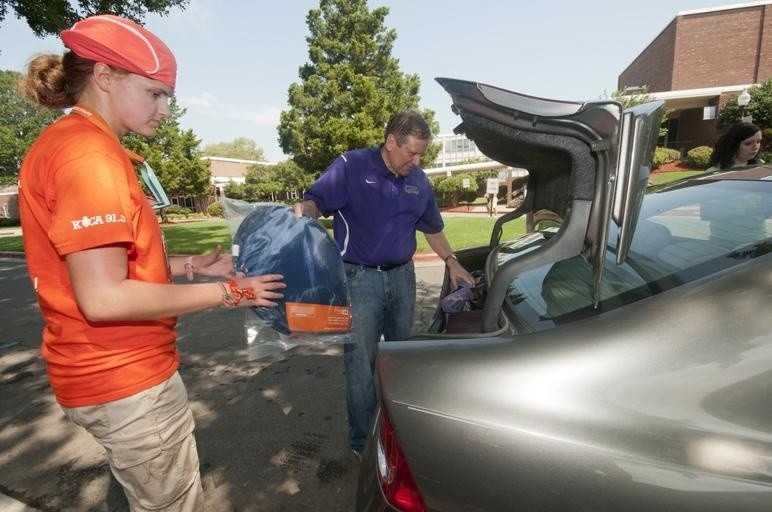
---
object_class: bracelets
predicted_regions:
[214,278,256,308]
[184,255,196,282]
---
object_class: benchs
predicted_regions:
[630,221,729,274]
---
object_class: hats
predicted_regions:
[56,14,177,89]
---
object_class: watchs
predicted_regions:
[444,253,458,269]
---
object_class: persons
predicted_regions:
[292,109,476,457]
[483,192,498,217]
[701,121,771,245]
[16,13,288,512]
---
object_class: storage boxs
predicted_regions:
[619,2,772,91]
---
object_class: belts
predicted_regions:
[339,258,414,273]
